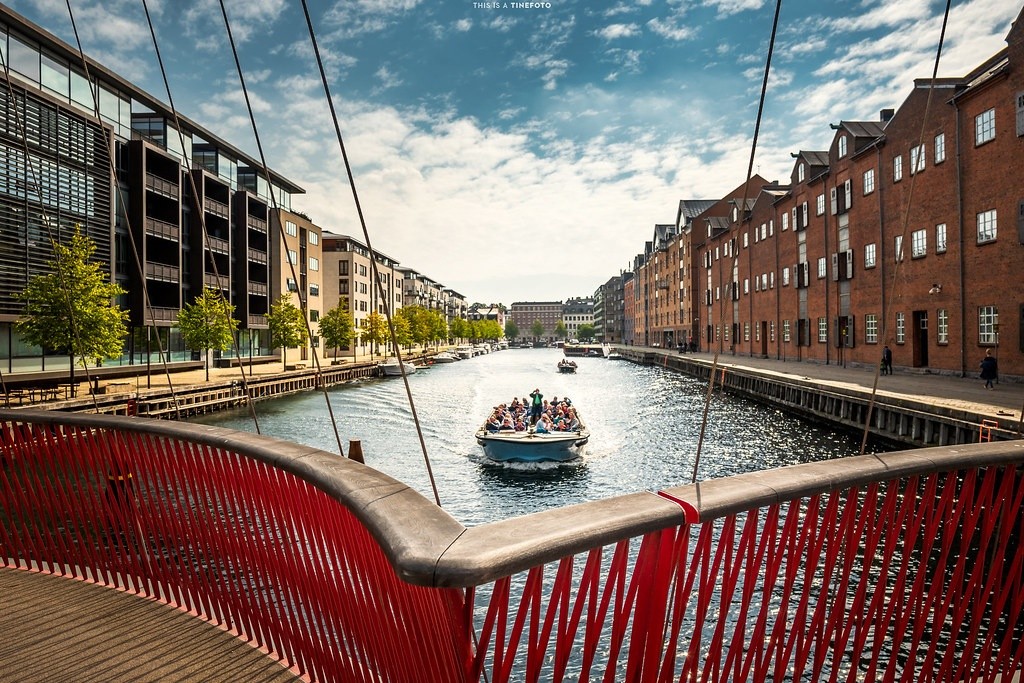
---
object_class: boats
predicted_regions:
[608,353,622,360]
[475,402,590,463]
[433,351,461,363]
[584,349,602,356]
[518,342,533,348]
[558,363,577,373]
[454,341,508,359]
[376,357,416,375]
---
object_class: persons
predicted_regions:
[420,334,699,436]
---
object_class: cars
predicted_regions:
[569,338,580,345]
